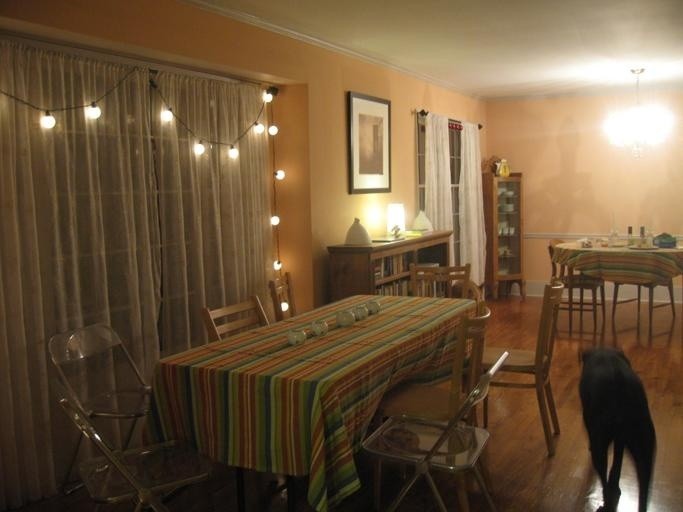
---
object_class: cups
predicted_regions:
[497,224,516,236]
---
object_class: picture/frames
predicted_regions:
[348,90,392,194]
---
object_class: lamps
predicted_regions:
[387,202,404,234]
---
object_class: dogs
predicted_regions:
[577,344,656,512]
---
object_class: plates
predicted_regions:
[611,242,626,246]
[501,204,515,213]
[630,245,660,250]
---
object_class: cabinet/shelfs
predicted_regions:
[483,172,525,302]
[327,231,452,301]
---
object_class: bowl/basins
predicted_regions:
[502,191,514,196]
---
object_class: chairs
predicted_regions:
[46,324,209,512]
[360,350,509,512]
[268,268,297,320]
[546,243,605,328]
[481,276,561,457]
[201,294,270,341]
[611,279,676,326]
[408,262,471,297]
[375,306,491,486]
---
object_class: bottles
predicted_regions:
[343,218,372,246]
[287,300,381,345]
[626,225,646,248]
[411,210,433,230]
[498,158,510,178]
